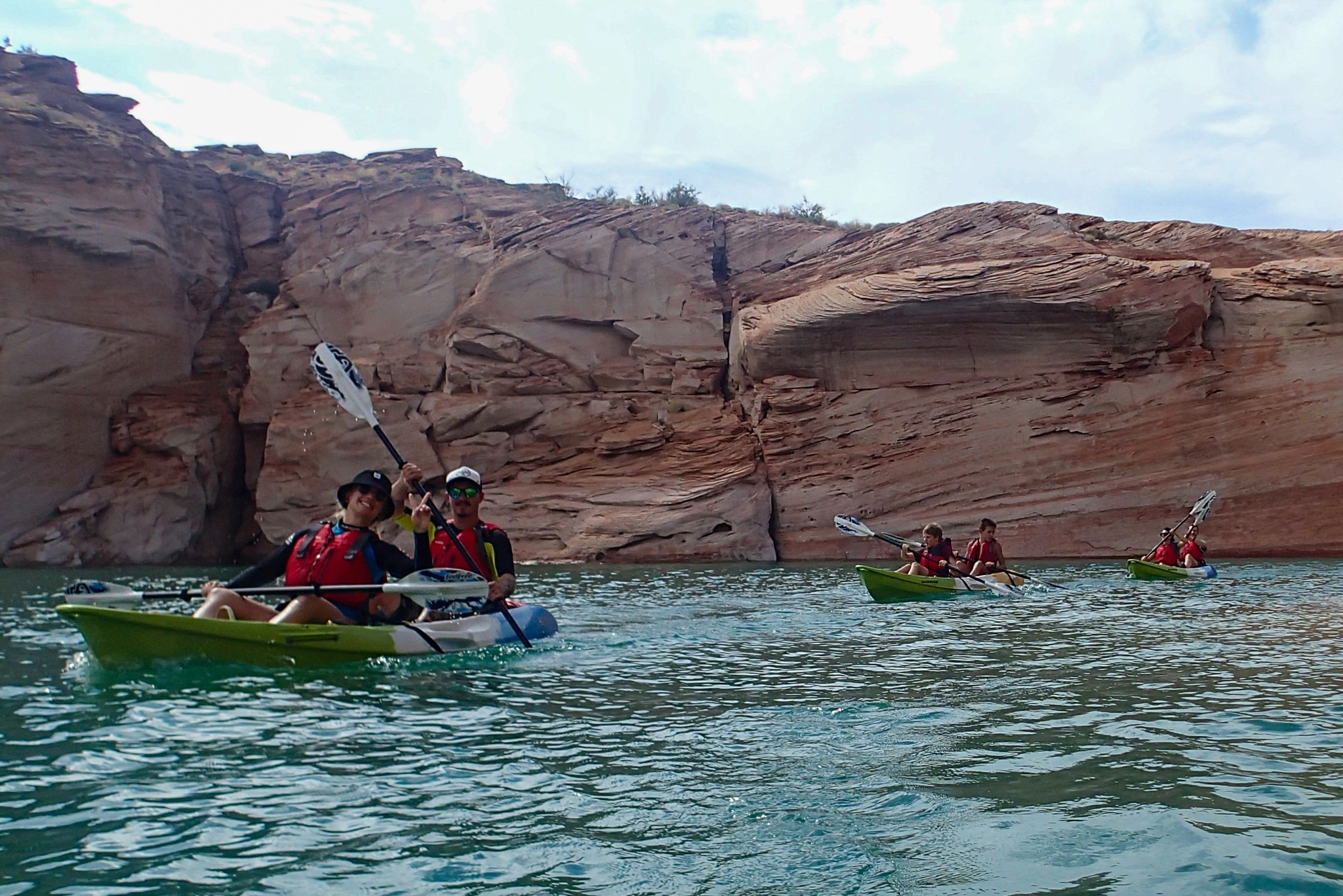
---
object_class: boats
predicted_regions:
[56,602,559,674]
[855,564,1025,604]
[1126,559,1218,581]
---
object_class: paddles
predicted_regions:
[1145,489,1217,558]
[833,514,1024,598]
[311,343,534,649]
[880,531,1070,591]
[1186,507,1211,537]
[64,567,488,615]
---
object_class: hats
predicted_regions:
[337,469,395,522]
[445,466,483,493]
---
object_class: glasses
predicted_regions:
[447,487,479,499]
[356,484,387,502]
[1160,532,1169,536]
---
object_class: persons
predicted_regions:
[1141,524,1207,568]
[368,462,516,624]
[193,470,431,625]
[895,518,1006,577]
